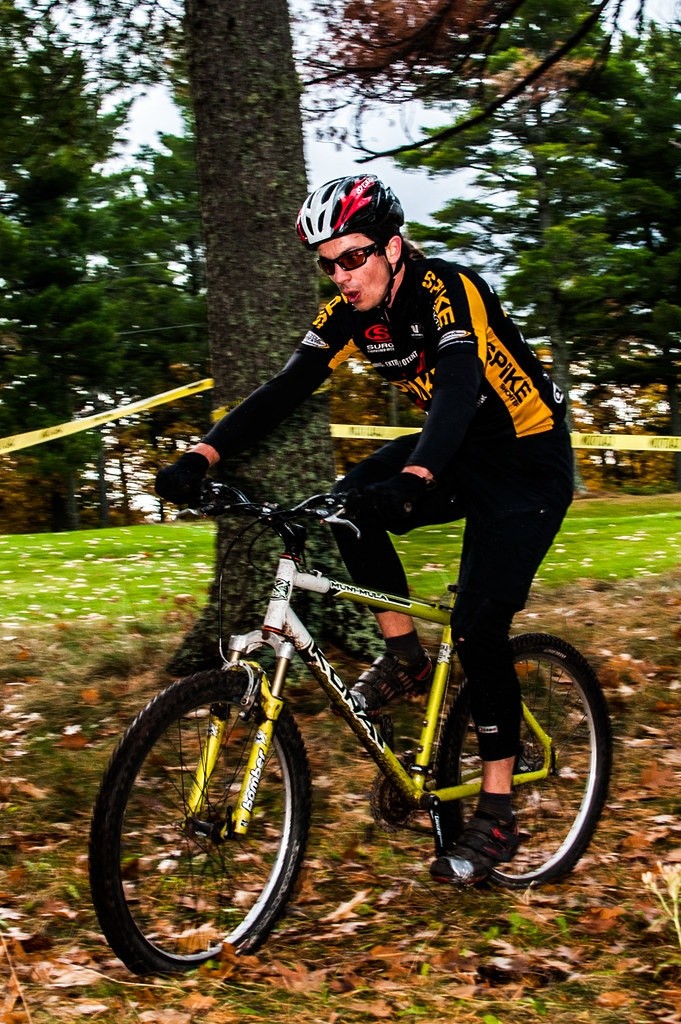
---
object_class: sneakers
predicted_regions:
[330,647,433,718]
[428,807,519,885]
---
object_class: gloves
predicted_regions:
[361,473,428,534]
[154,452,209,510]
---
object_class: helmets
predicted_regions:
[295,174,404,251]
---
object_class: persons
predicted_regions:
[155,174,573,883]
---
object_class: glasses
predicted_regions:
[317,243,377,275]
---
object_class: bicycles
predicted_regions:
[85,479,615,979]
